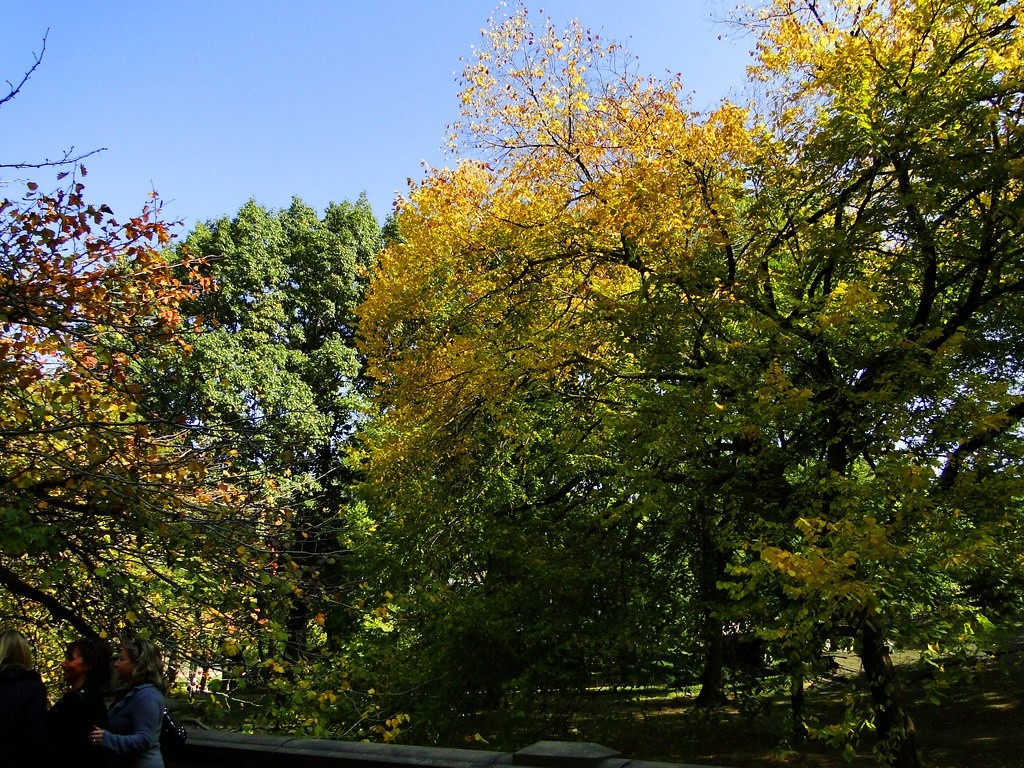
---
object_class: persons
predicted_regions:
[0,630,46,768]
[89,639,165,768]
[47,638,113,768]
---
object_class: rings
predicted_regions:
[93,739,95,742]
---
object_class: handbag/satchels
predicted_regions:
[159,709,187,750]
